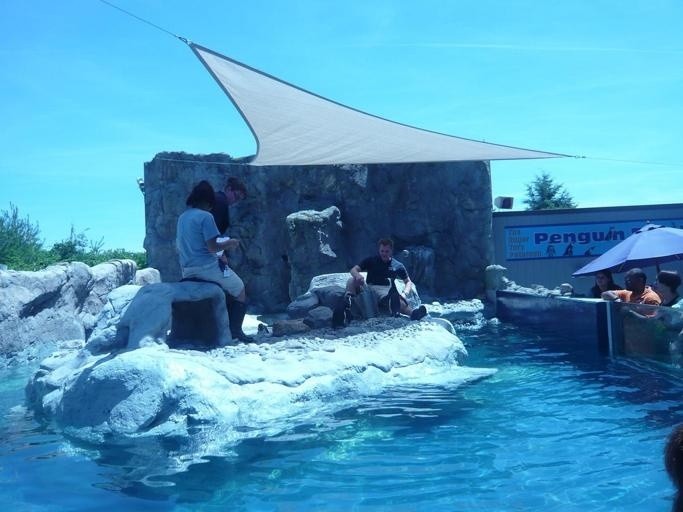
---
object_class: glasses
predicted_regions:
[234,191,240,200]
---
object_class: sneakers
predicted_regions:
[411,305,426,320]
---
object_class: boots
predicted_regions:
[228,300,253,342]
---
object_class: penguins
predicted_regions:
[332,290,358,330]
[256,323,270,335]
[410,304,427,321]
[380,276,409,318]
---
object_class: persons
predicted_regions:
[333,239,426,330]
[599,267,661,355]
[575,269,624,370]
[208,176,249,299]
[620,270,683,370]
[175,179,256,344]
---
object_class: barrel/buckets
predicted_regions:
[352,282,380,319]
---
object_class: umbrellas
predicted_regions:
[570,220,683,278]
